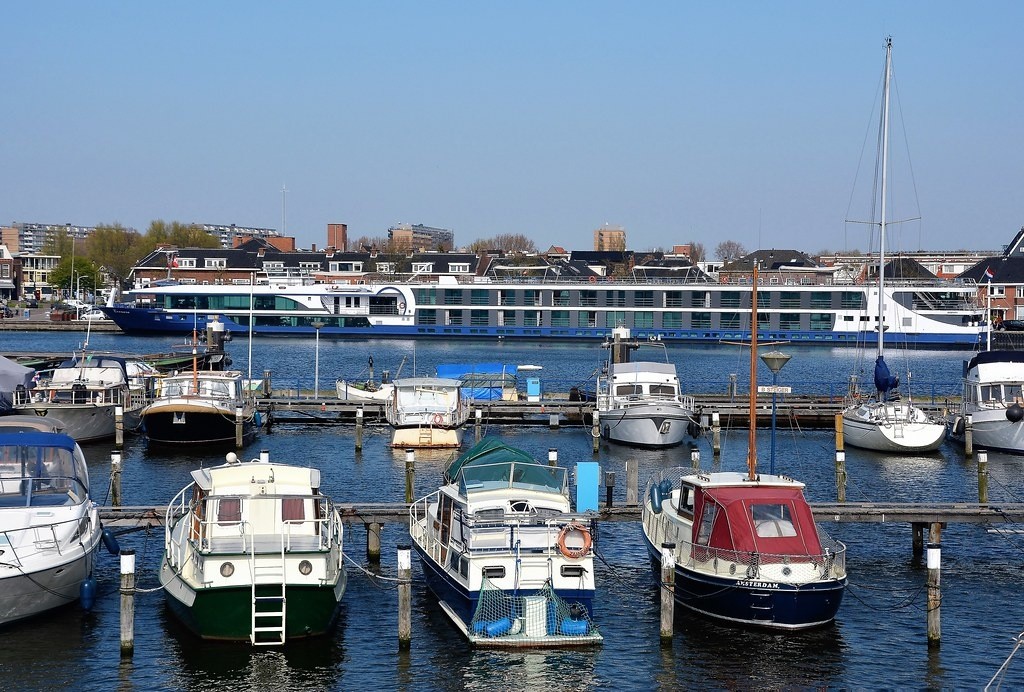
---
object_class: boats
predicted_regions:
[640,463,850,628]
[0,410,105,626]
[410,433,604,645]
[0,326,1023,462]
[154,448,348,649]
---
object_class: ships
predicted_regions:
[97,278,996,347]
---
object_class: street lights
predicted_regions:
[76,274,89,321]
[759,351,791,474]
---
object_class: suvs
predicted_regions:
[0,298,113,321]
[996,319,1024,331]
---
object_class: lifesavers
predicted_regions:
[399,302,406,309]
[589,276,596,284]
[434,414,443,425]
[606,276,614,283]
[722,276,728,283]
[558,522,592,559]
[855,277,864,285]
